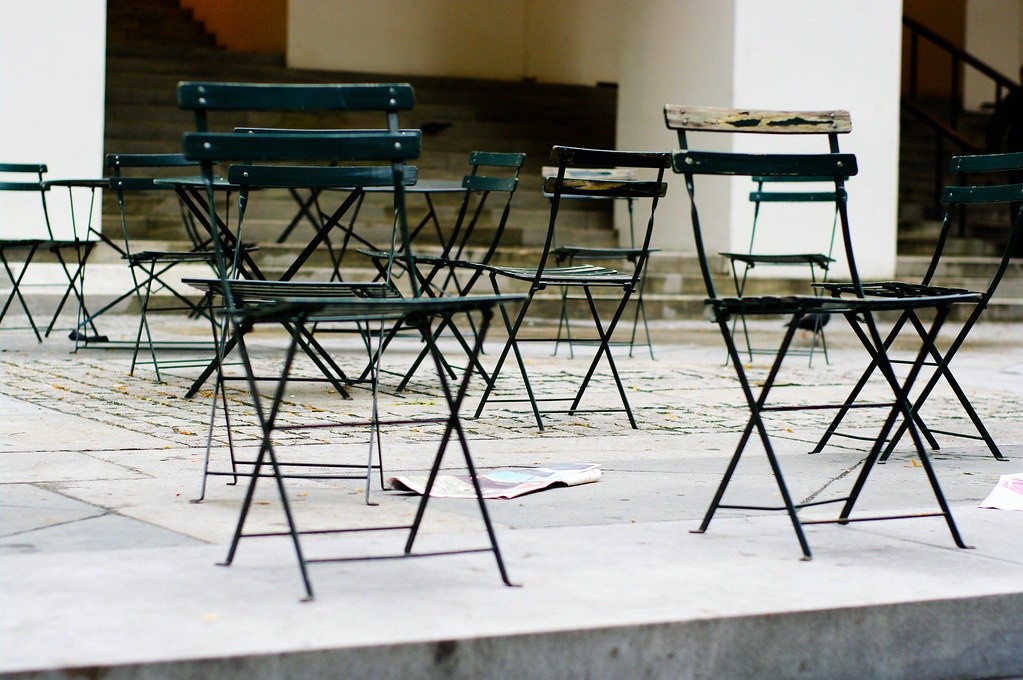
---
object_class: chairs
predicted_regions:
[0,80,1023,604]
